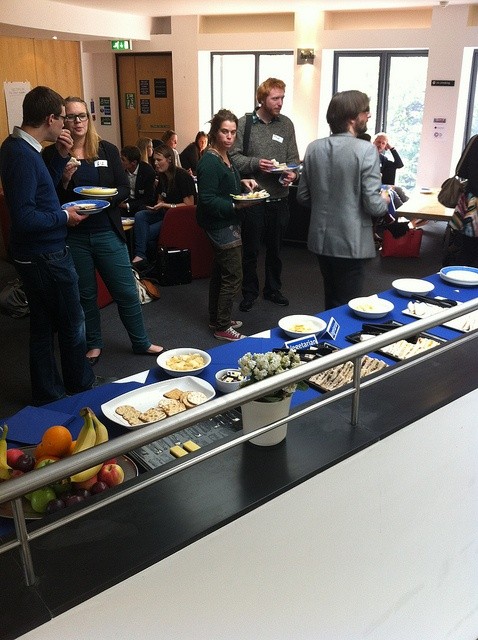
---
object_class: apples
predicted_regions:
[6,449,23,463]
[23,460,56,513]
[82,457,125,490]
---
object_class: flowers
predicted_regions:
[235,347,303,401]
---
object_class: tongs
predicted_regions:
[413,294,457,308]
[273,348,332,356]
[362,323,417,344]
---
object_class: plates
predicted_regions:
[290,341,342,393]
[0,446,138,521]
[440,266,478,286]
[278,315,327,340]
[156,348,211,376]
[273,166,300,173]
[348,297,393,320]
[100,376,216,432]
[127,409,243,470]
[74,186,117,200]
[402,296,478,333]
[122,219,135,226]
[391,278,435,298]
[62,199,110,215]
[346,320,448,362]
[234,192,268,202]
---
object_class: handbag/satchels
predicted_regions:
[438,134,478,207]
[379,227,423,259]
[142,280,161,300]
[149,247,192,285]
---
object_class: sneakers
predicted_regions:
[209,320,243,329]
[213,326,247,341]
[131,269,151,305]
[239,297,256,311]
[263,290,289,307]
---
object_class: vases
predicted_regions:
[240,395,291,447]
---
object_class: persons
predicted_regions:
[296,90,391,311]
[43,96,169,366]
[161,129,193,176]
[149,139,164,166]
[180,131,208,171]
[443,133,477,269]
[135,136,154,168]
[131,144,197,267]
[371,132,404,250]
[118,145,156,217]
[0,85,120,406]
[196,109,258,341]
[228,78,300,311]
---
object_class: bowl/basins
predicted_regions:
[215,369,244,392]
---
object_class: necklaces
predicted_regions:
[76,155,80,158]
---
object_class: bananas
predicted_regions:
[0,423,14,481]
[72,408,109,483]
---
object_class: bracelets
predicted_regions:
[170,203,177,209]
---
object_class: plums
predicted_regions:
[44,482,107,513]
[16,454,36,474]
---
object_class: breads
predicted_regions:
[381,336,440,360]
[308,355,392,392]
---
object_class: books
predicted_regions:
[380,184,409,213]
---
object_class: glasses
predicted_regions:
[52,113,68,123]
[66,113,89,122]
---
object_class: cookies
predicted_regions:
[169,444,185,460]
[184,439,199,453]
[113,389,206,426]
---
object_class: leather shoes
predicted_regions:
[85,347,102,365]
[134,345,168,356]
